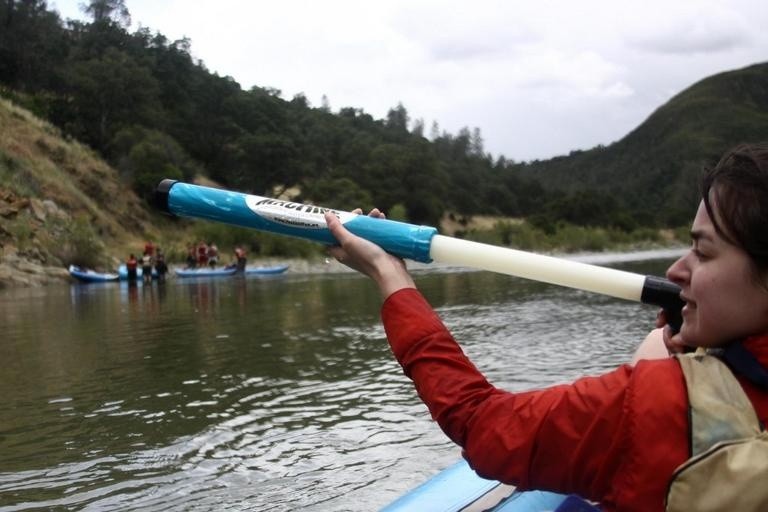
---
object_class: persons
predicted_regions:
[319,141,767,512]
[155,253,168,280]
[141,251,153,283]
[127,276,248,319]
[144,239,155,257]
[182,239,220,270]
[126,254,138,287]
[232,246,248,271]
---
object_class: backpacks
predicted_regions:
[665,348,767,512]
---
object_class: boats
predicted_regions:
[68,262,290,282]
